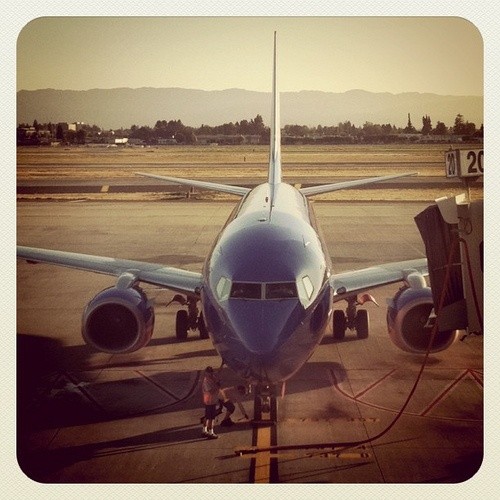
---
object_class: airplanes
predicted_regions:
[16,29,462,428]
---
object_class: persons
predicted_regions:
[200,367,221,439]
[200,383,250,427]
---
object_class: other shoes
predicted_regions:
[208,434,219,440]
[202,431,209,437]
[220,417,235,425]
